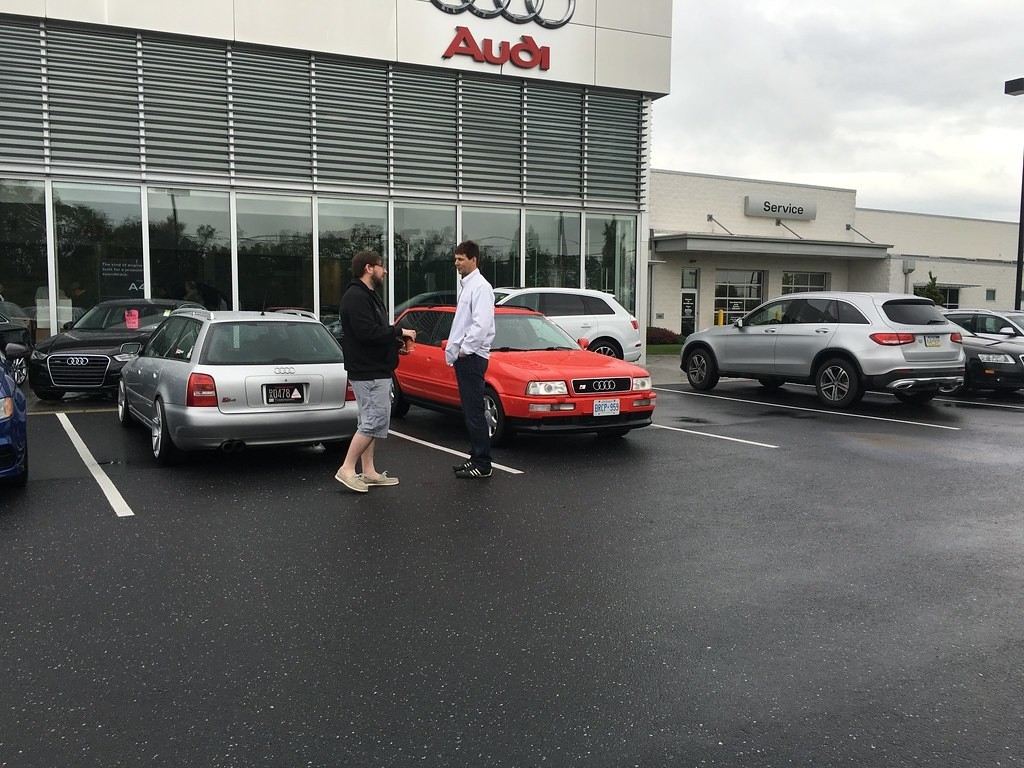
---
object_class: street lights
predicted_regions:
[1004,77,1024,312]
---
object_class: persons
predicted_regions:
[215,293,228,311]
[183,280,204,305]
[335,251,416,492]
[444,242,496,479]
[76,284,97,310]
[0,284,7,301]
[34,286,66,305]
[159,284,171,299]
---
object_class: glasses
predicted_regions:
[375,263,386,270]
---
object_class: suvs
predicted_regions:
[936,309,1024,398]
[490,287,642,366]
[681,291,968,410]
[28,299,209,401]
[116,309,361,462]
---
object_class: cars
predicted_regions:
[388,307,657,448]
[0,343,34,486]
[392,290,458,321]
[0,300,31,385]
[266,306,344,341]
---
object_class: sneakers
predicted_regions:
[335,467,368,493]
[453,458,472,471]
[455,465,492,479]
[360,470,399,486]
